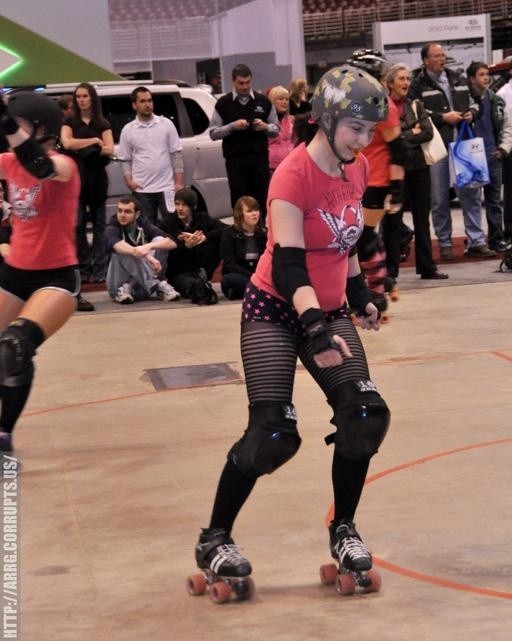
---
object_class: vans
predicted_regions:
[1,79,236,233]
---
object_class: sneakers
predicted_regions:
[155,279,181,302]
[400,229,512,261]
[81,275,90,284]
[114,283,134,304]
[93,276,105,283]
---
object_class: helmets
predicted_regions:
[310,64,389,123]
[345,47,390,82]
[6,90,63,139]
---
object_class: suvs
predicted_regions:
[487,55,512,94]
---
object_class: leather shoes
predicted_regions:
[420,268,449,280]
[77,294,95,310]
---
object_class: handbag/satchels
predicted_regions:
[409,99,448,168]
[447,117,490,191]
[188,274,219,305]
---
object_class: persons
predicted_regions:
[194,65,392,576]
[0,83,83,482]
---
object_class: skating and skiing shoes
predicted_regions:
[320,519,383,595]
[0,431,25,478]
[347,266,400,326]
[189,525,256,603]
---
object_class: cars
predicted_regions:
[444,56,465,74]
